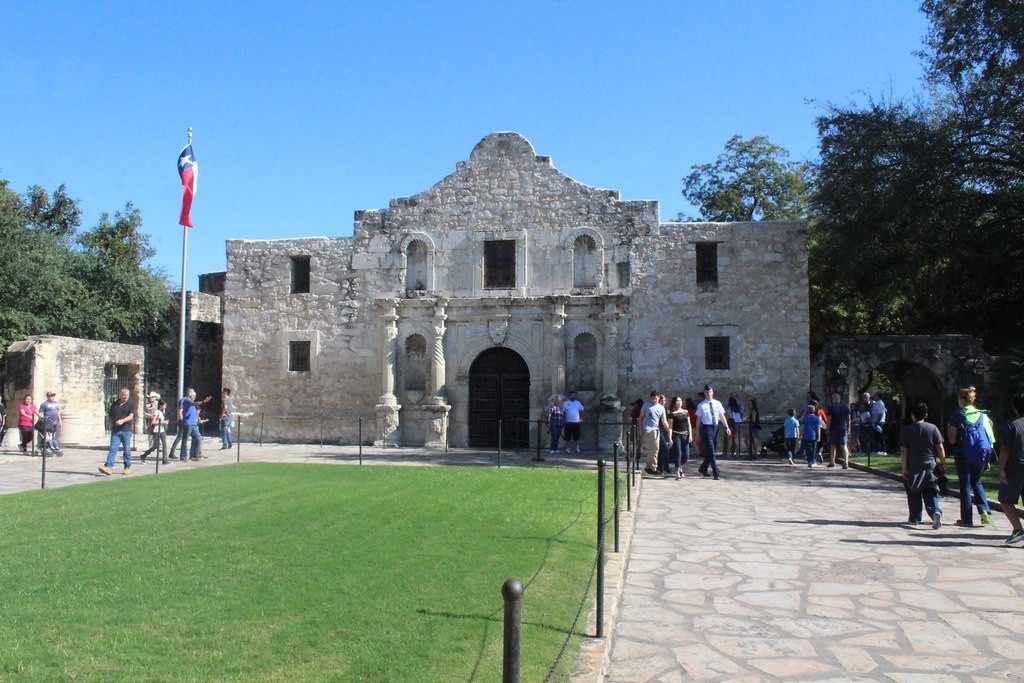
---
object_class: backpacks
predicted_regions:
[957,411,991,467]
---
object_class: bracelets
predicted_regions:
[725,426,729,429]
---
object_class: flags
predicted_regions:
[177,144,198,229]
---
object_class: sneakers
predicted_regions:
[956,519,973,526]
[1006,528,1024,544]
[980,514,991,524]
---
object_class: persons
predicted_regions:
[826,393,851,469]
[695,384,731,480]
[948,386,992,526]
[18,391,62,455]
[998,394,1024,543]
[799,405,821,467]
[795,391,827,463]
[784,408,800,466]
[140,391,169,465]
[899,403,946,530]
[684,390,762,459]
[0,396,6,433]
[169,387,212,462]
[630,390,672,476]
[850,390,887,457]
[547,391,584,454]
[667,396,693,481]
[99,387,134,475]
[219,388,232,450]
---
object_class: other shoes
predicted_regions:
[228,445,233,449]
[908,520,919,524]
[98,466,112,475]
[18,444,24,451]
[932,511,942,530]
[140,455,147,464]
[566,448,570,453]
[180,458,191,462]
[219,447,228,451]
[576,447,581,453]
[169,453,178,459]
[550,450,555,453]
[644,451,896,480]
[23,451,30,456]
[189,456,205,461]
[555,449,560,453]
[123,469,130,475]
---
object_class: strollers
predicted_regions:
[761,426,788,458]
[34,418,65,458]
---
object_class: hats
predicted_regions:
[703,384,712,390]
[146,391,160,399]
[46,391,56,398]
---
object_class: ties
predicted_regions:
[710,402,716,427]
